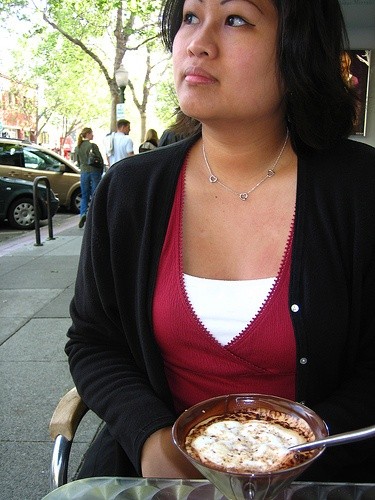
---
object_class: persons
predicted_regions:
[139,129,159,152]
[339,52,360,128]
[74,127,103,228]
[62,0,375,484]
[104,119,133,169]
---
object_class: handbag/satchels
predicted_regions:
[87,143,101,168]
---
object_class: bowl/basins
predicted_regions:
[171,393,328,500]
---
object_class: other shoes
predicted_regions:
[79,214,87,228]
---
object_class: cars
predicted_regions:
[0,175,61,230]
[0,137,91,214]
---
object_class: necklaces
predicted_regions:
[202,126,289,200]
